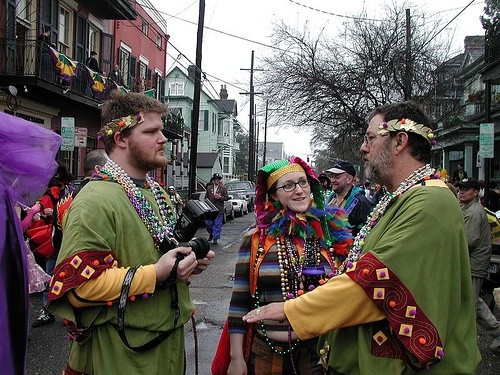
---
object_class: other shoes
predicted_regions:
[208,236,212,241]
[214,240,217,244]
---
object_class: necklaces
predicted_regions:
[338,163,435,277]
[103,158,177,253]
[255,233,337,375]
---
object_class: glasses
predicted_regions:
[363,133,378,146]
[458,186,469,191]
[274,179,310,192]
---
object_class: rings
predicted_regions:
[257,311,260,314]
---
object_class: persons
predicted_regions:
[321,160,387,235]
[243,104,472,375]
[441,160,500,359]
[48,90,217,375]
[226,158,355,375]
[0,26,127,375]
[169,173,229,244]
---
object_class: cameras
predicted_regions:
[158,198,219,260]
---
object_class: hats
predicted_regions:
[317,174,331,186]
[326,161,356,177]
[454,177,479,192]
[211,173,222,180]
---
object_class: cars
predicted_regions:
[188,179,256,224]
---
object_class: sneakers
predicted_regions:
[32,312,54,327]
